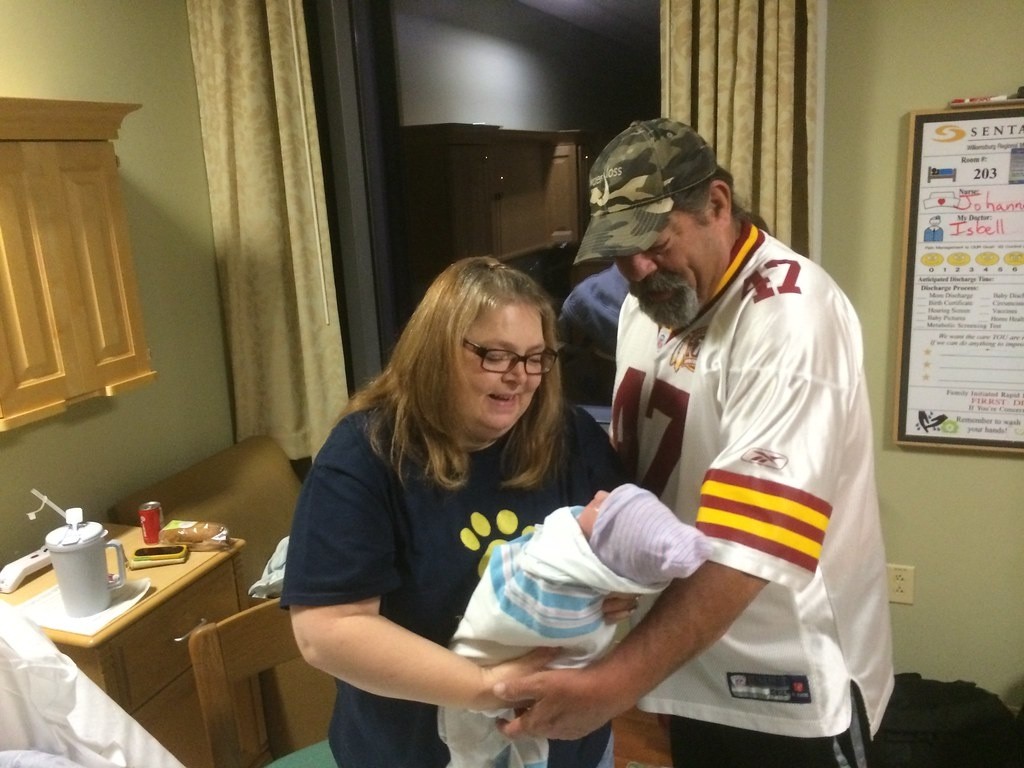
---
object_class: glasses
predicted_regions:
[463,337,559,374]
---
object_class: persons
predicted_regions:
[491,118,896,768]
[438,483,716,768]
[279,255,640,768]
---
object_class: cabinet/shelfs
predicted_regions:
[0,521,274,768]
[402,123,603,388]
[0,97,159,431]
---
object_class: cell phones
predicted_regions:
[133,545,187,560]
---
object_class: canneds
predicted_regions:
[138,501,165,546]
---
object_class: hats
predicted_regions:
[572,118,718,267]
[589,483,708,584]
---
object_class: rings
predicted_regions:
[635,595,639,601]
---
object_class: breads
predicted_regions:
[157,520,225,552]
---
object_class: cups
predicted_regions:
[45,508,127,619]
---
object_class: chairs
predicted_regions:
[188,598,337,768]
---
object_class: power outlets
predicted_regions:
[887,563,915,605]
